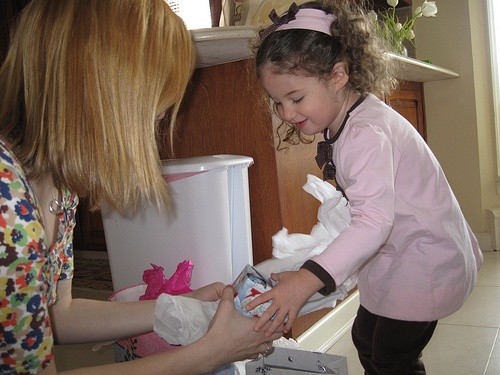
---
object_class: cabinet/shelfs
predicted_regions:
[264,56,428,342]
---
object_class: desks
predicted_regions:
[180,25,462,84]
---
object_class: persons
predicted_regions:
[1,1,289,375]
[226,0,487,375]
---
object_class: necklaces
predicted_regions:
[47,176,63,215]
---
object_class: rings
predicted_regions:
[262,344,270,357]
